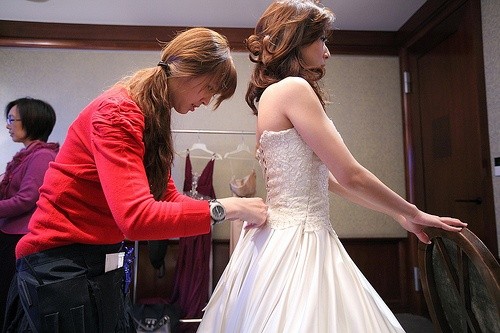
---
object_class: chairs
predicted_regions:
[417,226,500,333]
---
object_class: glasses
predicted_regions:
[6,118,22,125]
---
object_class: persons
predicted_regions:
[0,96,60,333]
[197,0,469,333]
[14,27,268,333]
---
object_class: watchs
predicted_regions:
[209,199,227,225]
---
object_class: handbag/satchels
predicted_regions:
[126,304,180,333]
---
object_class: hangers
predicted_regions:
[225,130,256,160]
[175,130,223,161]
